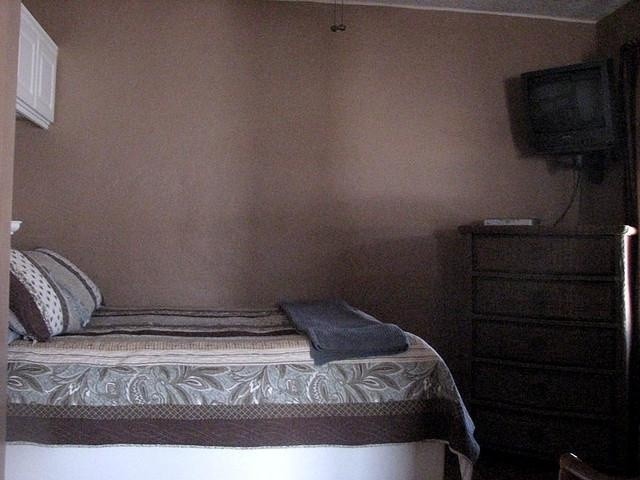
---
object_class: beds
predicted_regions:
[6,306,445,479]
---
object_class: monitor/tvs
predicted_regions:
[521,57,620,154]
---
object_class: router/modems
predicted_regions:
[484,217,540,226]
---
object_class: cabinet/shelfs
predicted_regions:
[458,226,638,480]
[16,3,59,128]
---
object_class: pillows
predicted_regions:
[11,247,103,343]
